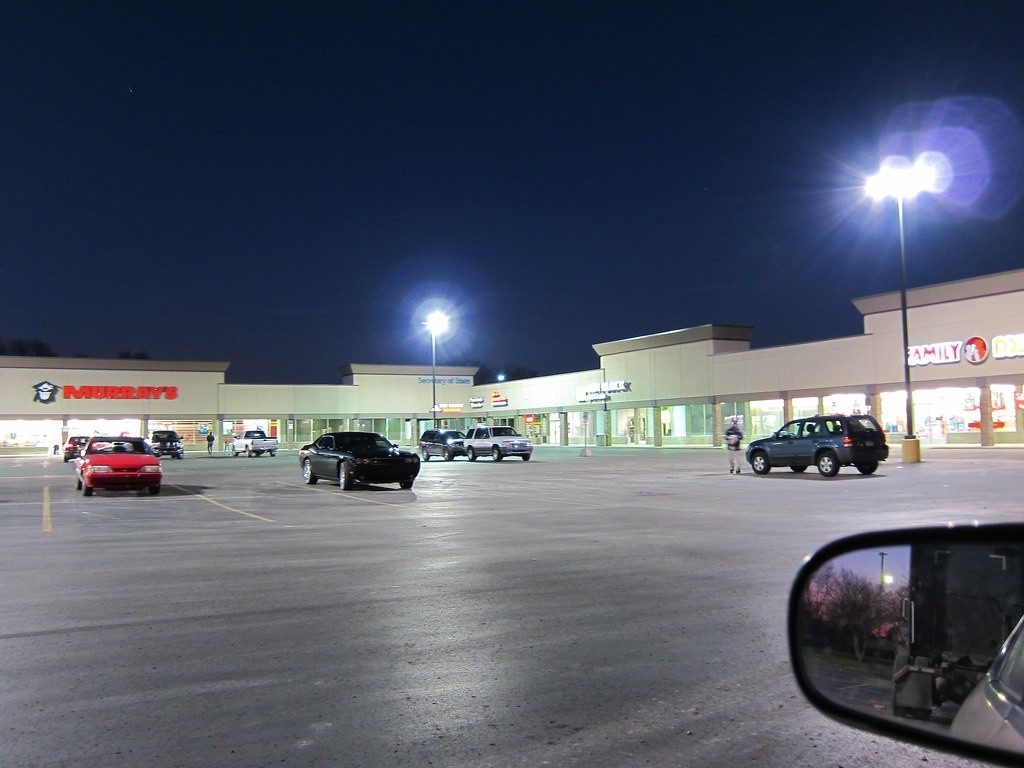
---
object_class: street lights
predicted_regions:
[864,163,932,464]
[425,310,450,429]
[878,551,887,654]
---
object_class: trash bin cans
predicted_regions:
[595,432,607,446]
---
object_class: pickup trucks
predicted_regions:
[230,430,279,458]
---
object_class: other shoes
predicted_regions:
[736,469,740,474]
[730,469,733,473]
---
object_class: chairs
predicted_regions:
[807,424,815,437]
[833,425,840,431]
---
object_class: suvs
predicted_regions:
[417,425,533,461]
[744,411,889,477]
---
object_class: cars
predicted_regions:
[144,430,185,459]
[62,436,164,496]
[298,432,420,491]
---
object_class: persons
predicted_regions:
[725,418,743,474]
[206,432,214,455]
